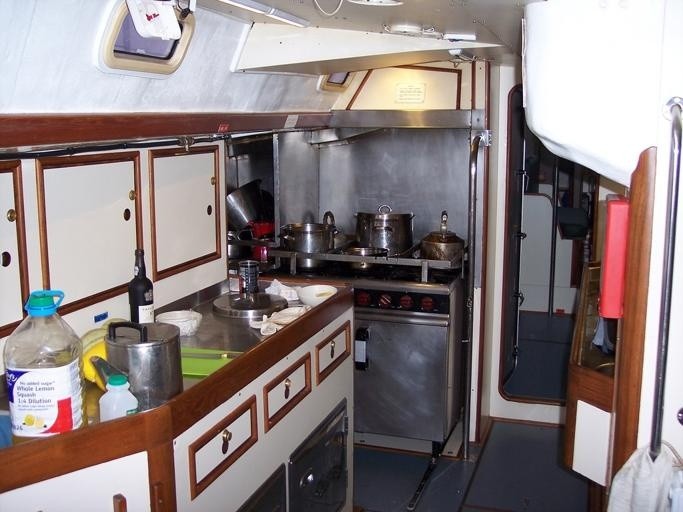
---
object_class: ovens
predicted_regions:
[353,289,450,444]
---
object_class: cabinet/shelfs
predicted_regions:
[144,136,225,308]
[29,147,151,336]
[0,145,29,337]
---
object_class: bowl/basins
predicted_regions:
[343,247,387,270]
[156,310,203,337]
[296,284,337,309]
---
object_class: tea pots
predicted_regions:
[420,210,465,271]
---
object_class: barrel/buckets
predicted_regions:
[4,291,85,442]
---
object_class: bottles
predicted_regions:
[98,376,138,421]
[127,250,157,323]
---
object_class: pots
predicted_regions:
[352,204,416,258]
[278,209,342,270]
[104,321,184,411]
